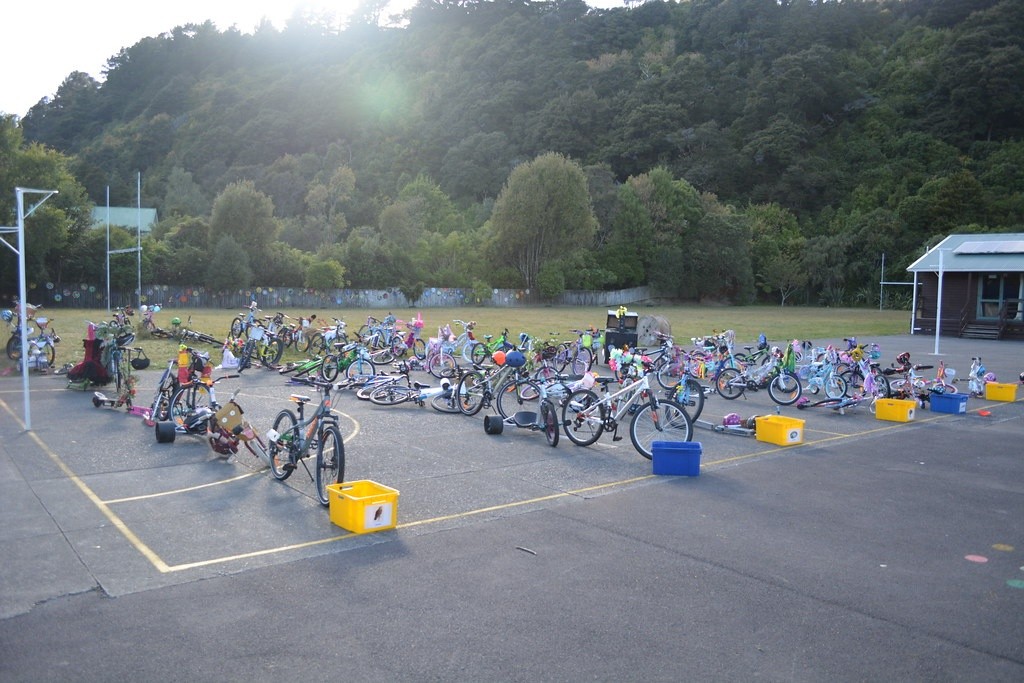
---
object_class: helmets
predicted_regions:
[2,309,13,322]
[172,318,181,324]
[126,309,134,316]
[184,407,214,435]
[208,428,238,455]
[50,334,61,341]
[113,333,135,346]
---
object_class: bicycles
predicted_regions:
[0,300,973,508]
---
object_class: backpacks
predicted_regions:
[131,351,150,369]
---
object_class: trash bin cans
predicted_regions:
[605,310,638,364]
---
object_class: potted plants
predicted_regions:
[907,295,925,317]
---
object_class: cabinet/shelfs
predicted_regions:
[604,309,638,363]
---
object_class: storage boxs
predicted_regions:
[755,414,806,446]
[985,381,1018,403]
[650,441,703,478]
[326,479,400,535]
[929,392,969,415]
[875,398,916,422]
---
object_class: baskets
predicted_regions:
[249,326,264,340]
[302,320,310,327]
